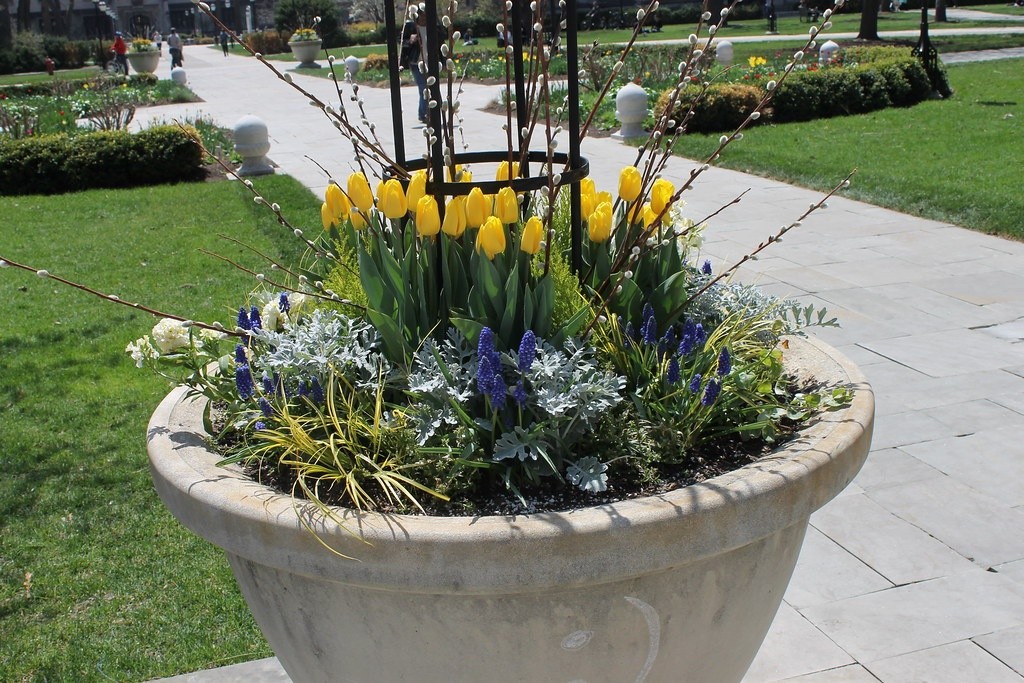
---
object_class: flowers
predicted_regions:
[0,0,861,564]
[129,38,156,53]
[289,29,319,41]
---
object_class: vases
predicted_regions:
[287,39,323,68]
[144,329,876,683]
[124,50,162,73]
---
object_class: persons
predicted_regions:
[399,2,444,124]
[154,31,162,57]
[167,28,184,71]
[220,29,228,57]
[464,28,478,46]
[108,31,128,76]
